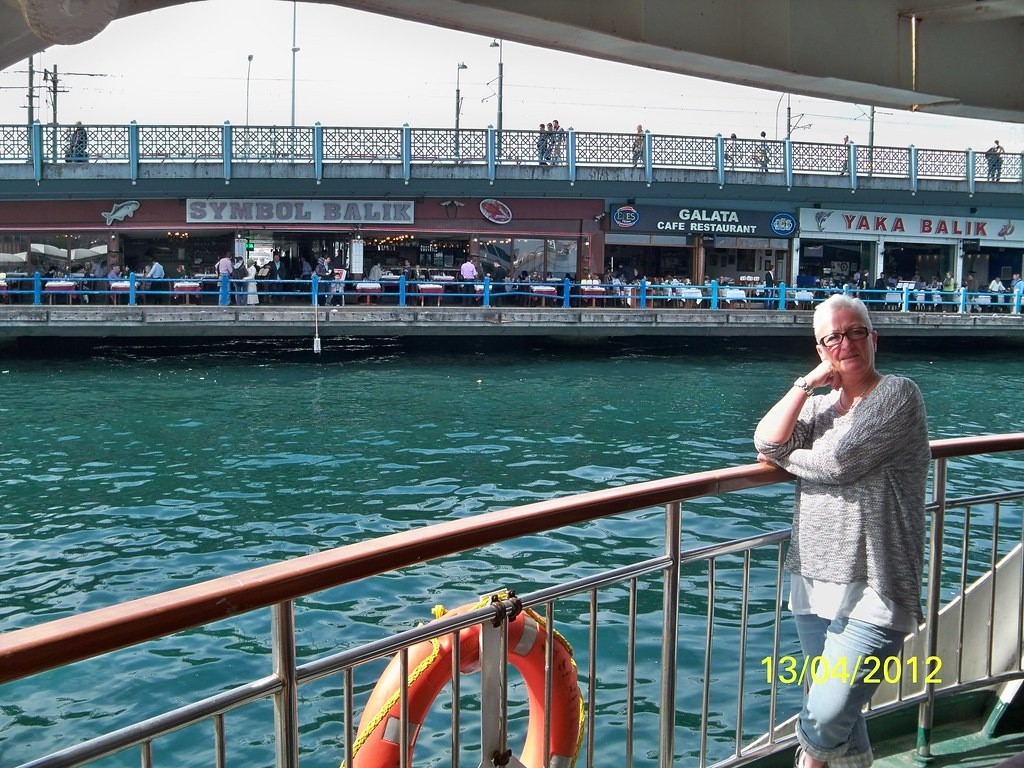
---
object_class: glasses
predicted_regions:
[817,326,873,347]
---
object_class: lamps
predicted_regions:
[594,211,605,223]
[813,203,821,208]
[626,193,636,205]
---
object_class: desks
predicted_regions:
[110,281,143,305]
[0,272,7,301]
[914,293,942,311]
[417,284,445,306]
[173,281,204,305]
[193,271,218,279]
[623,286,634,307]
[6,271,29,304]
[885,292,902,310]
[793,291,815,310]
[721,289,747,309]
[429,274,456,289]
[578,286,608,308]
[355,283,381,306]
[979,296,1004,303]
[45,280,77,305]
[680,288,703,308]
[382,274,401,280]
[530,286,558,307]
[631,287,655,308]
[135,272,148,278]
[473,284,493,307]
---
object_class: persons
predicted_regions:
[985,139,1005,182]
[537,120,565,166]
[839,135,853,175]
[70,121,86,163]
[755,131,769,172]
[37,248,1024,313]
[754,293,932,768]
[632,124,646,167]
[728,133,740,170]
[5,267,24,305]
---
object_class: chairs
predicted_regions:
[705,282,719,308]
[325,268,349,305]
[523,286,535,306]
[166,276,185,304]
[660,284,676,307]
[607,287,619,307]
[101,272,121,305]
[9,274,26,303]
[457,279,469,304]
[73,281,84,304]
[85,275,97,302]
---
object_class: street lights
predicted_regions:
[489,37,503,157]
[245,54,254,126]
[868,110,894,176]
[456,61,468,157]
[291,47,301,163]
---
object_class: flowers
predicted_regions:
[176,264,188,274]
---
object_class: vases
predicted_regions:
[183,274,189,283]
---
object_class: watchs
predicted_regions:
[794,376,814,399]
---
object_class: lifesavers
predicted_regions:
[352,601,582,768]
